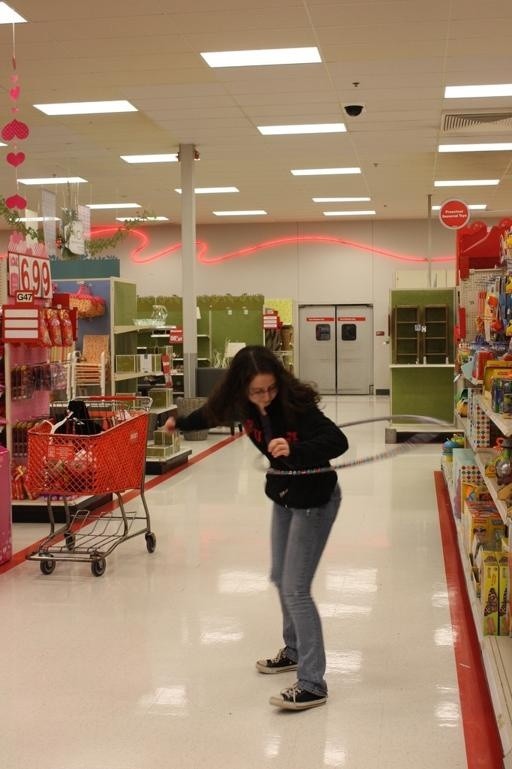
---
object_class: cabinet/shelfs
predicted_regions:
[389,287,466,433]
[137,300,243,435]
[434,219,512,768]
[1,265,109,524]
[264,296,295,378]
[54,277,194,476]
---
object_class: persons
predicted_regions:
[165,345,350,712]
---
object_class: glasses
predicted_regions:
[248,384,279,396]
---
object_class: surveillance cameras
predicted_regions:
[344,97,364,116]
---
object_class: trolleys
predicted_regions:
[22,393,160,578]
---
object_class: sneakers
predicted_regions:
[268,683,327,710]
[255,649,297,673]
